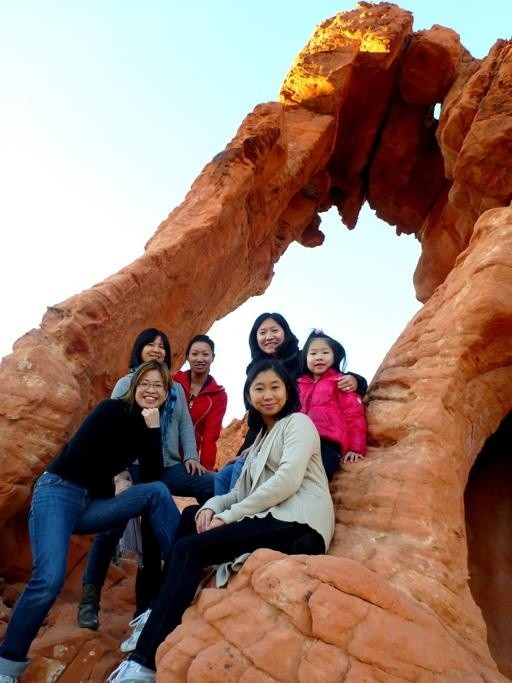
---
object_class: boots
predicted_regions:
[77,574,104,629]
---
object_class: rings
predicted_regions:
[346,386,351,391]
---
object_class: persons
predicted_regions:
[0,358,217,683]
[98,356,338,683]
[213,329,369,496]
[76,328,215,629]
[113,333,229,619]
[235,312,369,461]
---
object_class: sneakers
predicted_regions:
[106,659,156,683]
[120,608,152,653]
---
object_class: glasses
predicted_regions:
[137,379,164,392]
